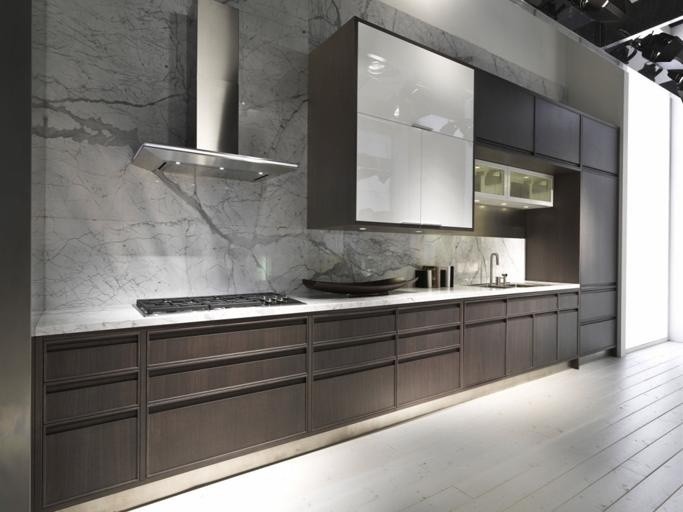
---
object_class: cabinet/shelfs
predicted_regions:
[307,16,476,232]
[476,68,534,171]
[534,93,581,180]
[396,299,463,421]
[579,113,619,359]
[474,159,555,210]
[146,313,309,483]
[30,325,146,512]
[309,304,396,452]
[463,291,579,390]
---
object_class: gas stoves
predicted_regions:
[136,291,306,317]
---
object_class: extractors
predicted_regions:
[131,0,299,183]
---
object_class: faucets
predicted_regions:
[488,252,499,284]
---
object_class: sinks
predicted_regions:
[470,280,551,289]
